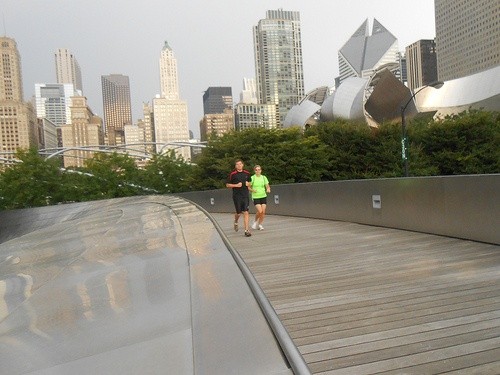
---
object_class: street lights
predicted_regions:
[402,81,445,162]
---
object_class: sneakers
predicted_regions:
[245,231,251,237]
[258,224,265,230]
[251,219,256,229]
[233,220,238,231]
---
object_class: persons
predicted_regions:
[225,159,252,237]
[247,165,271,230]
